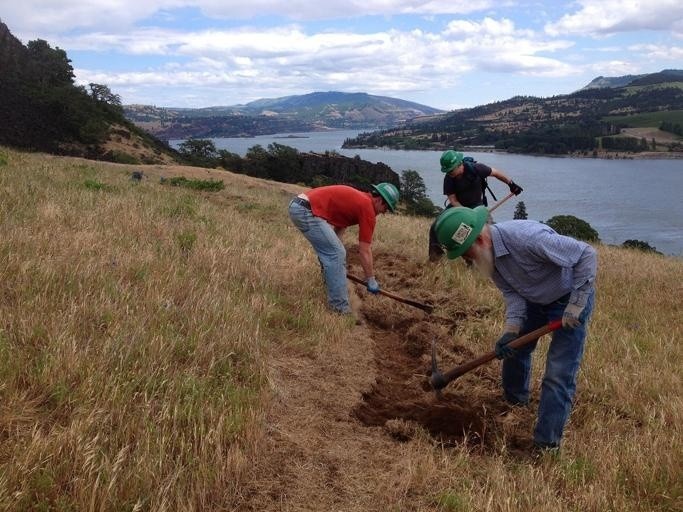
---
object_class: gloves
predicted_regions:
[368,276,378,292]
[508,180,524,197]
[495,332,519,361]
[561,302,584,329]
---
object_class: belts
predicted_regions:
[292,196,311,209]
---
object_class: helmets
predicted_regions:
[371,182,400,213]
[435,205,489,260]
[440,149,464,173]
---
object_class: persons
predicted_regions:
[429,150,523,269]
[434,206,597,464]
[288,182,399,325]
